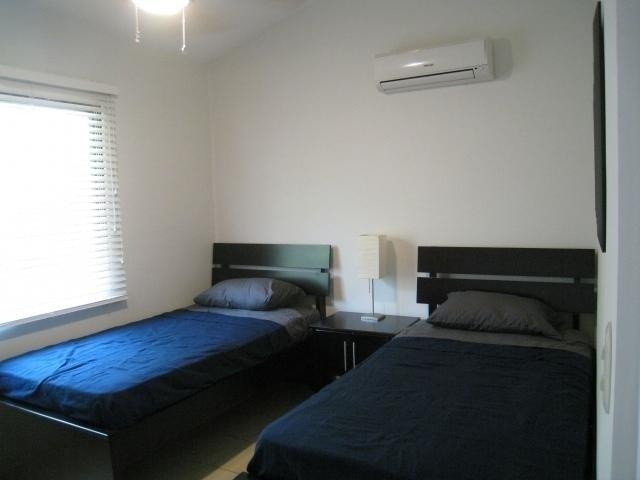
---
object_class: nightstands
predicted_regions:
[306,311,421,381]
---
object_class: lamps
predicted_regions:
[358,234,388,321]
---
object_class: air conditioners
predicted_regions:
[374,38,496,95]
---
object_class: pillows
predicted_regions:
[193,276,309,312]
[428,291,563,341]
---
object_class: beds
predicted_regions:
[235,245,596,478]
[1,244,333,478]
